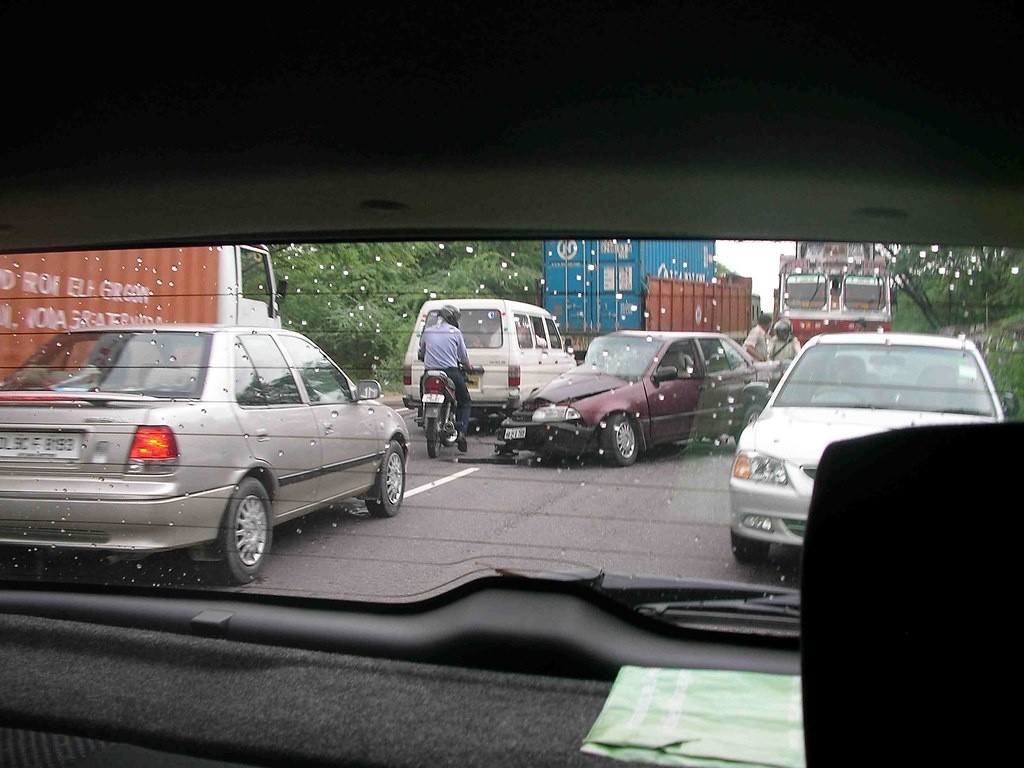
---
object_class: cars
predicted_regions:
[0,324,410,588]
[728,333,1005,566]
[493,330,785,468]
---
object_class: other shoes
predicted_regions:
[457,432,467,452]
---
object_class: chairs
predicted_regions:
[916,364,958,393]
[827,356,872,397]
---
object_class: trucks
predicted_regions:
[542,241,764,360]
[1,244,290,385]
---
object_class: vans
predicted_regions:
[401,299,578,433]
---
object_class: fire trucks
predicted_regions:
[771,241,891,349]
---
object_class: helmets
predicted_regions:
[773,318,793,342]
[437,305,461,327]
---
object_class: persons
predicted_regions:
[854,318,866,332]
[475,323,547,347]
[742,315,773,363]
[770,319,800,360]
[417,305,477,452]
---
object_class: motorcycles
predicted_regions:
[414,363,472,459]
[732,357,790,446]
